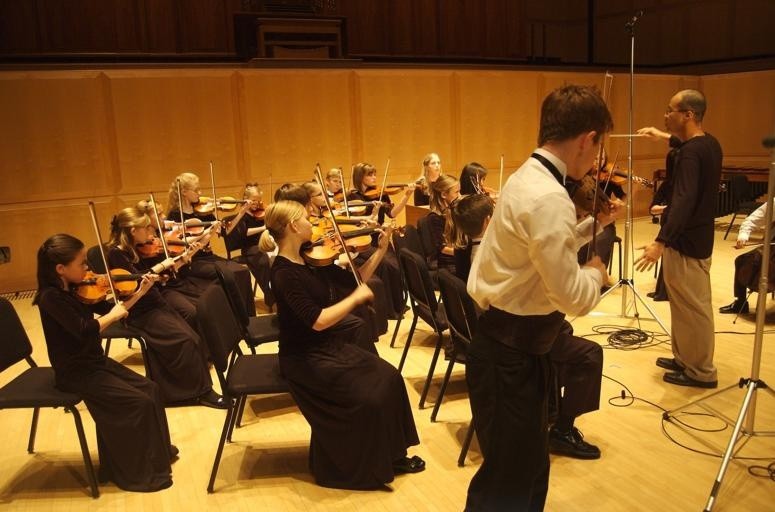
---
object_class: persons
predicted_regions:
[273,181,378,360]
[456,162,489,199]
[257,201,425,494]
[34,234,180,495]
[649,149,681,300]
[234,186,275,310]
[633,89,724,392]
[346,164,416,217]
[167,173,256,325]
[134,197,243,370]
[418,174,466,289]
[324,167,345,196]
[413,151,442,205]
[306,178,408,318]
[467,86,615,511]
[97,205,233,409]
[592,144,629,289]
[720,196,775,312]
[453,195,604,460]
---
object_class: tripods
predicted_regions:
[662,151,775,512]
[566,32,672,339]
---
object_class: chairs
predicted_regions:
[397,247,448,409]
[390,224,440,348]
[0,296,100,500]
[100,321,150,378]
[197,283,296,494]
[222,214,242,260]
[430,268,483,466]
[214,259,280,380]
[87,242,110,274]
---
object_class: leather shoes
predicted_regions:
[663,371,718,389]
[199,390,235,408]
[654,294,669,301]
[656,356,684,371]
[548,426,601,458]
[393,453,424,472]
[720,300,749,314]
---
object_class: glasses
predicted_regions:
[666,108,685,114]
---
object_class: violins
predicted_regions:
[365,183,428,198]
[159,218,232,238]
[321,199,395,218]
[73,268,169,304]
[592,162,654,188]
[302,226,406,266]
[194,196,257,216]
[310,216,366,228]
[136,234,211,257]
